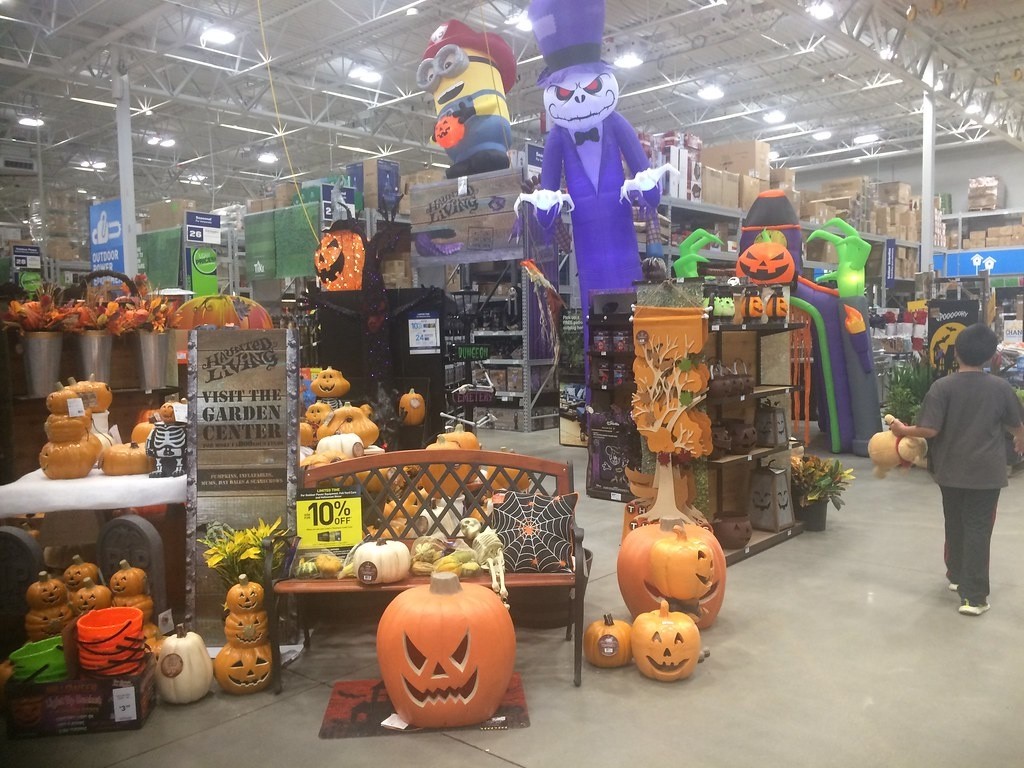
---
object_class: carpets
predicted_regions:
[318,672,531,739]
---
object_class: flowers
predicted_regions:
[791,454,856,511]
[0,274,183,333]
[197,515,290,611]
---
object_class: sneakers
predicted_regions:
[943,572,961,592]
[958,595,992,616]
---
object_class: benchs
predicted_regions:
[261,449,585,694]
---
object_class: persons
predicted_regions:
[889,324,1024,615]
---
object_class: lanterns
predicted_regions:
[25,554,164,658]
[705,284,794,552]
[212,574,272,696]
[583,518,725,682]
[375,573,517,728]
[39,373,112,479]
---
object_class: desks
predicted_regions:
[0,460,187,532]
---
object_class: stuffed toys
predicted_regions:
[417,20,515,178]
[868,414,929,479]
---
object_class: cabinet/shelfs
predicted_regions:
[349,166,1024,568]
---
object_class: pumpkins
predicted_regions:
[154,621,214,704]
[299,423,531,537]
[99,404,161,477]
[294,537,481,585]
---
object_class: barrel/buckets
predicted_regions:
[9,635,71,684]
[76,606,148,677]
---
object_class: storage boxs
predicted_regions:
[149,199,196,230]
[52,246,73,260]
[6,240,32,255]
[252,141,1024,432]
[5,653,156,739]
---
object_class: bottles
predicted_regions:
[449,343,458,363]
[446,314,464,336]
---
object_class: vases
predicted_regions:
[128,329,169,390]
[23,332,63,397]
[79,330,112,383]
[222,611,291,642]
[793,497,826,531]
[505,547,592,629]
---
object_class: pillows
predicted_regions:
[492,489,578,573]
